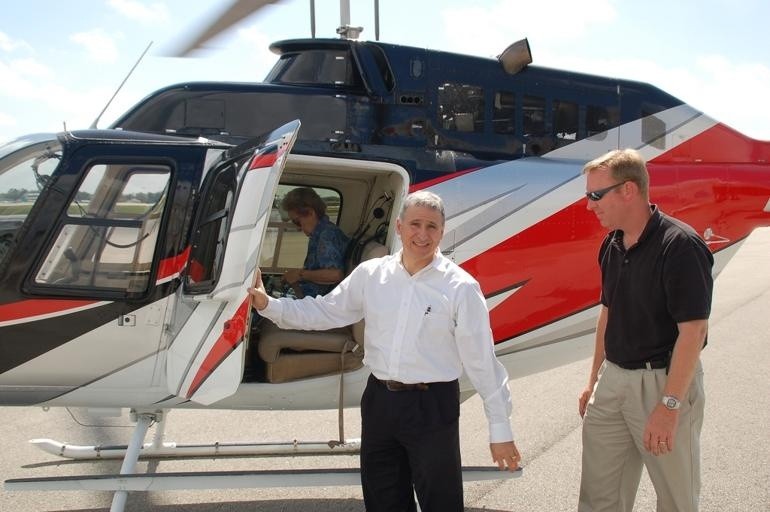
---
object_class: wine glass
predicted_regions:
[658,440,667,444]
[512,455,517,460]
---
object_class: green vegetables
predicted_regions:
[0,0,769,511]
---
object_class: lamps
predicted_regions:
[258,240,389,381]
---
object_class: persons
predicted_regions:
[245,187,522,512]
[251,185,351,329]
[574,146,715,512]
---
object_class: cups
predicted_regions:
[587,183,624,201]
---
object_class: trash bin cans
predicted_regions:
[661,393,681,411]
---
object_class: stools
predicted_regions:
[375,379,429,391]
[604,351,671,370]
